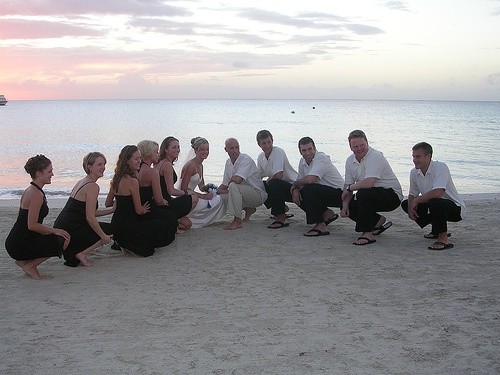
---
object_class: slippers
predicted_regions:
[270,213,293,219]
[353,237,376,245]
[267,220,290,228]
[424,232,452,238]
[428,242,454,250]
[372,221,392,236]
[304,229,330,236]
[325,214,339,226]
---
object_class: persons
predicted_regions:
[4,154,71,279]
[290,137,344,237]
[53,151,117,266]
[174,137,226,229]
[151,137,199,230]
[401,141,467,251]
[134,140,178,240]
[256,130,300,229]
[217,138,268,230]
[339,130,403,245]
[105,145,155,257]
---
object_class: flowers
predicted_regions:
[203,183,219,209]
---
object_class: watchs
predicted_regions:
[346,184,352,192]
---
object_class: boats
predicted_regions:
[0,94,8,106]
